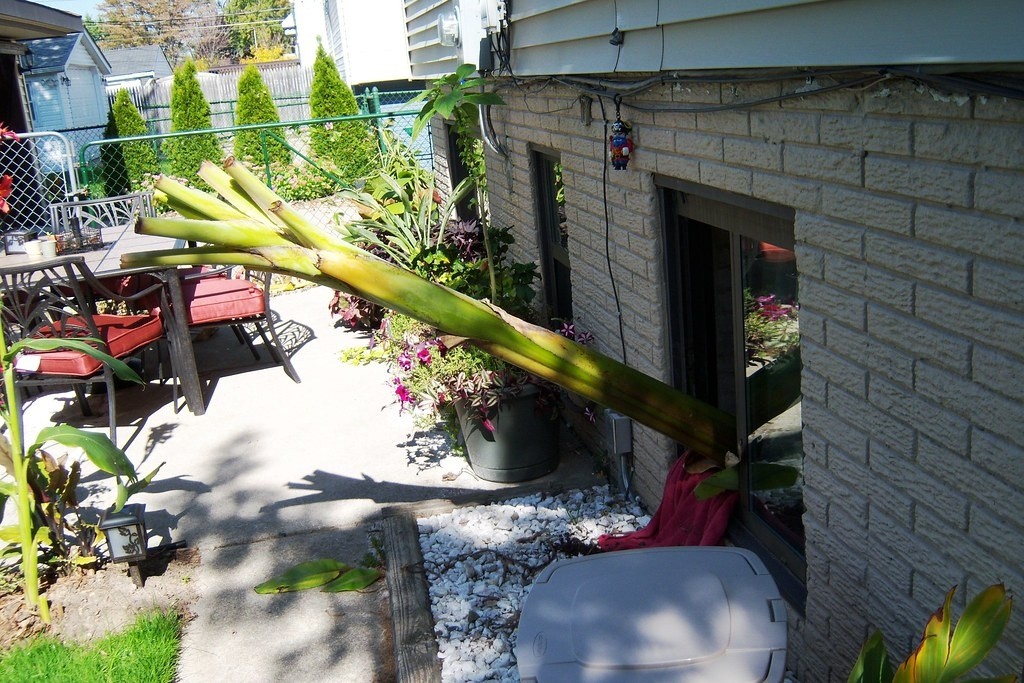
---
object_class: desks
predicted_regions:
[0,216,205,415]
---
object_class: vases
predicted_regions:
[455,384,560,482]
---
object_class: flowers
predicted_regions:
[392,316,596,431]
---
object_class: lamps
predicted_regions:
[62,76,71,86]
[98,504,149,564]
[437,5,462,48]
[18,47,34,75]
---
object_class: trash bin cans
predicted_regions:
[515,546,787,683]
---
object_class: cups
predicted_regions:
[23,239,42,255]
[39,241,57,257]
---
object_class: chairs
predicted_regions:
[0,191,301,448]
